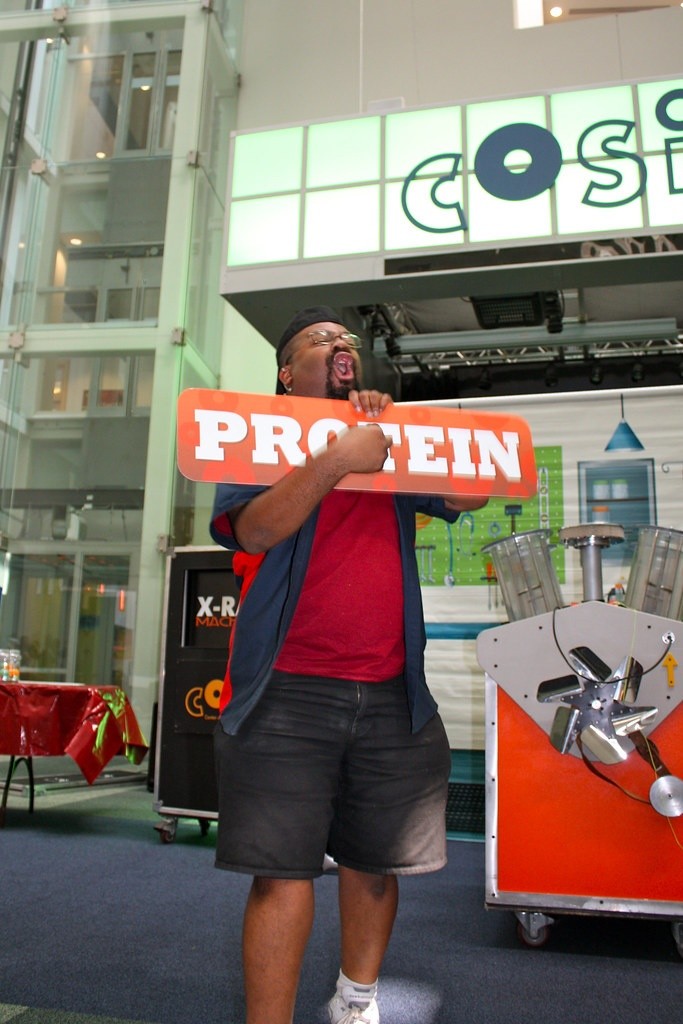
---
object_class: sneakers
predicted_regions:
[327,985,380,1024]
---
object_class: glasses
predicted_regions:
[285,329,364,366]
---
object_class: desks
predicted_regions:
[0,682,149,820]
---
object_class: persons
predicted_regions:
[207,304,490,1024]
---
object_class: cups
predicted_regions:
[0,649,21,683]
